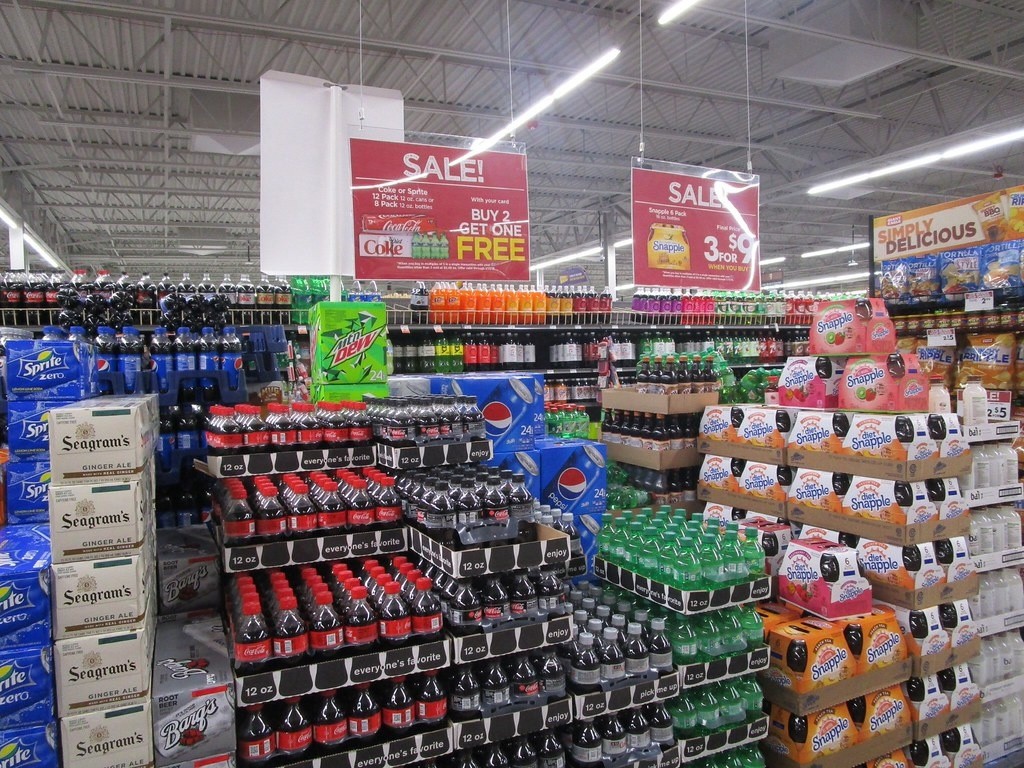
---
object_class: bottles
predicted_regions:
[0,266,1024,766]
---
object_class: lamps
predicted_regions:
[612,238,632,249]
[801,241,871,260]
[807,128,1024,195]
[531,246,603,271]
[657,0,698,25]
[0,206,17,229]
[760,257,785,268]
[448,48,623,166]
[24,233,58,269]
[762,270,884,291]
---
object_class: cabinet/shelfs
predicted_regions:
[1,301,1024,768]
